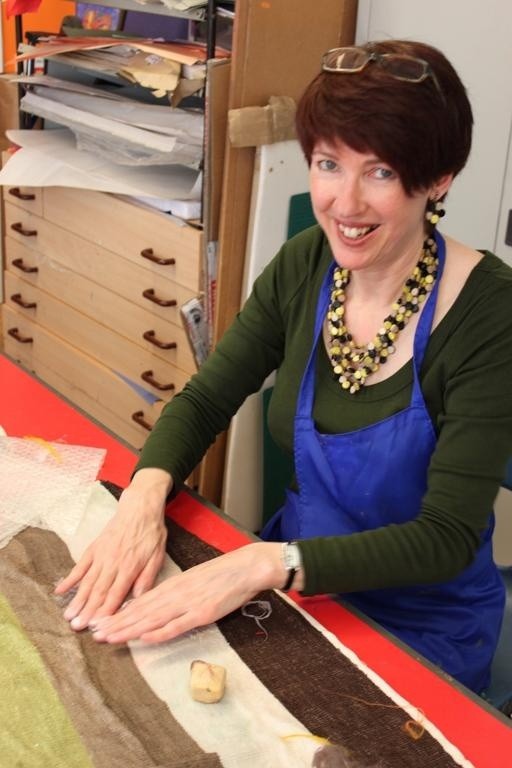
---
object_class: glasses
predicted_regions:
[320,46,442,100]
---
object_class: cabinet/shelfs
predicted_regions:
[0,0,357,504]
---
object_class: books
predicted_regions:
[0,1,236,221]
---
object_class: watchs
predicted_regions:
[280,540,301,590]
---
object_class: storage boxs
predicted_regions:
[72,0,197,43]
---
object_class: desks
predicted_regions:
[0,352,512,768]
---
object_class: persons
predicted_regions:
[50,29,512,694]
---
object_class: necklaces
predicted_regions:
[326,235,440,395]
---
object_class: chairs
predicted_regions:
[488,487,512,715]
